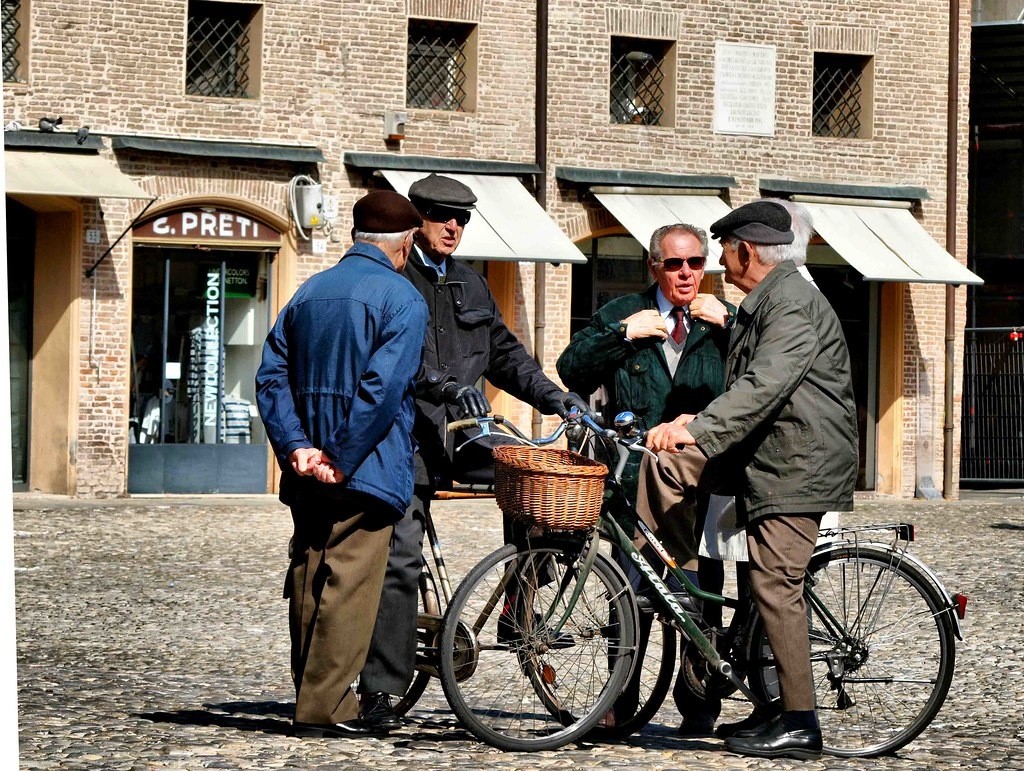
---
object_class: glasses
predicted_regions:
[413,202,471,225]
[651,256,706,271]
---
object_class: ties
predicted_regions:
[669,306,688,345]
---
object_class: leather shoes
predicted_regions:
[498,612,576,649]
[595,707,634,728]
[724,716,824,760]
[716,713,779,740]
[360,691,401,729]
[677,716,715,738]
[293,718,390,738]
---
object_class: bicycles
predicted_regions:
[341,406,677,743]
[435,407,968,757]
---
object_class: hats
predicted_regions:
[710,201,794,245]
[409,173,478,209]
[353,189,424,233]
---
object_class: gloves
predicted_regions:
[444,383,492,418]
[540,390,592,420]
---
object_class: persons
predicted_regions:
[641,201,855,764]
[562,225,747,738]
[254,188,428,737]
[345,172,596,731]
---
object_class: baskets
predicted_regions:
[493,444,608,529]
